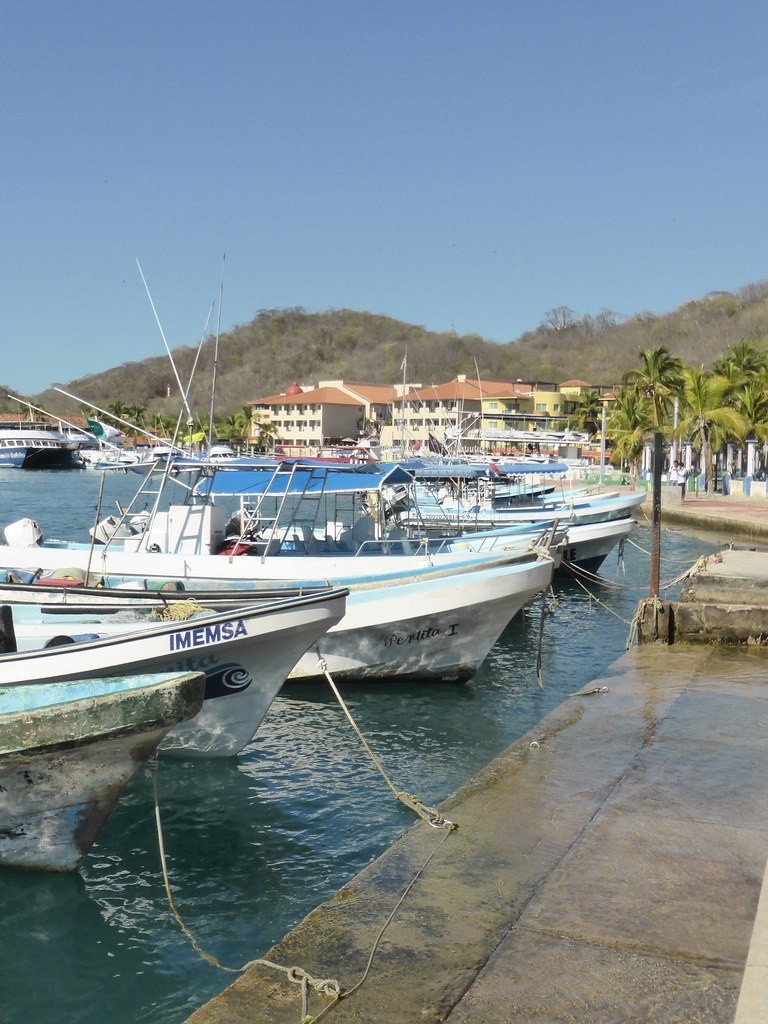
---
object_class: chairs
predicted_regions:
[292,517,413,553]
[468,505,480,513]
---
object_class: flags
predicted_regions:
[401,355,407,369]
[86,418,125,438]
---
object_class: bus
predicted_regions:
[272,443,370,465]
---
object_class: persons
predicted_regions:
[669,460,678,486]
[733,463,736,474]
[675,463,688,500]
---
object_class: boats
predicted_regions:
[0,242,650,879]
[0,420,72,468]
[0,669,208,873]
